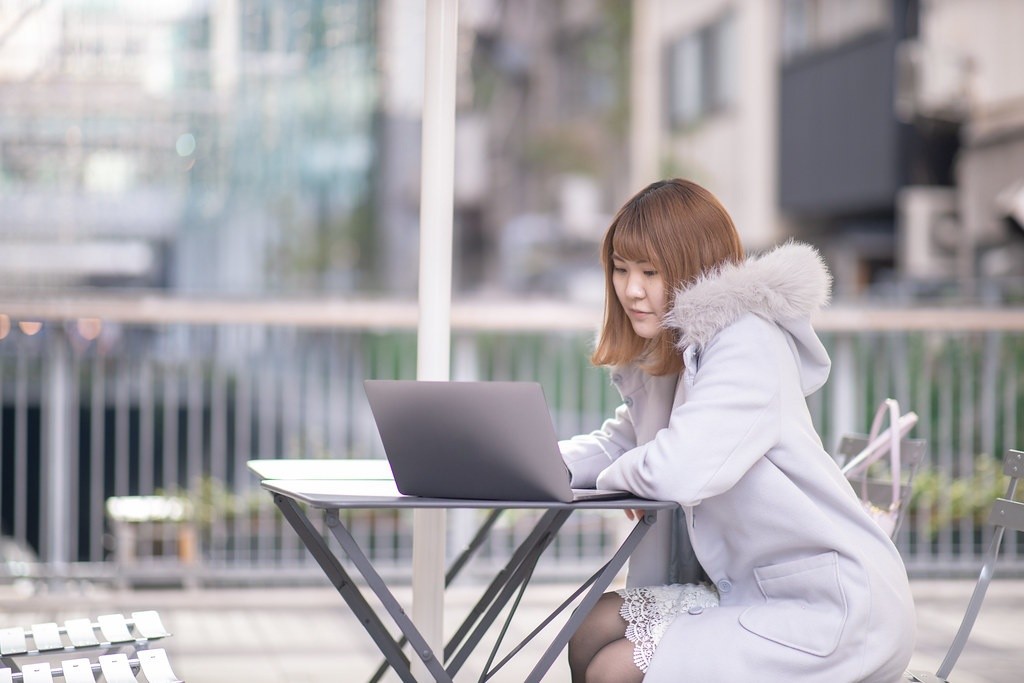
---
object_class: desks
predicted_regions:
[247,459,680,683]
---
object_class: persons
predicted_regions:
[557,179,917,683]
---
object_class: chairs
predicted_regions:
[899,449,1024,683]
[839,432,928,541]
[0,610,187,683]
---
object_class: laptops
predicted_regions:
[360,379,636,503]
